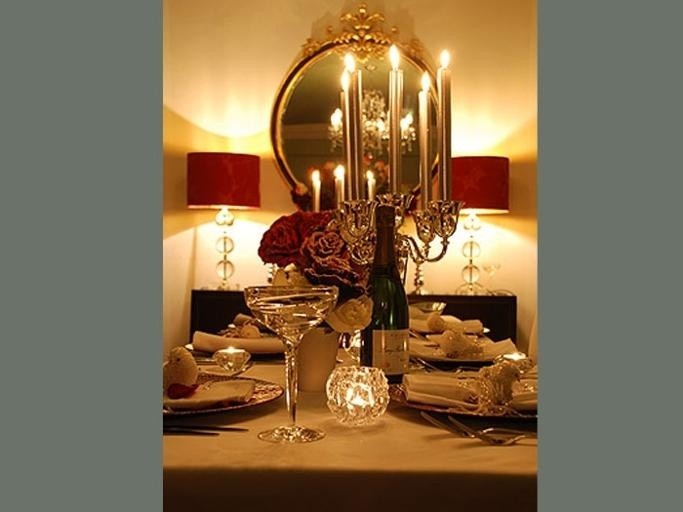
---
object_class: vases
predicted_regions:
[297,327,337,394]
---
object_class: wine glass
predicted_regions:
[245,285,338,442]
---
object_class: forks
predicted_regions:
[447,416,537,444]
[420,411,536,436]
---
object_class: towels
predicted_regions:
[409,319,484,334]
[402,372,538,409]
[408,338,526,359]
[164,381,255,409]
[192,331,285,355]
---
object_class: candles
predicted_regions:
[310,44,450,213]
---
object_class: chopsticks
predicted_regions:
[164,423,249,437]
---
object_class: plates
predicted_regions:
[161,372,284,415]
[185,343,277,355]
[411,322,490,334]
[410,336,503,361]
[388,382,536,418]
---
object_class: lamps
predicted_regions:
[452,157,509,295]
[187,152,261,290]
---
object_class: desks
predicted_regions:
[191,290,516,347]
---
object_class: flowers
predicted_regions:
[258,210,408,335]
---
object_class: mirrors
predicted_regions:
[272,7,440,213]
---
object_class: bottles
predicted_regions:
[361,207,409,383]
